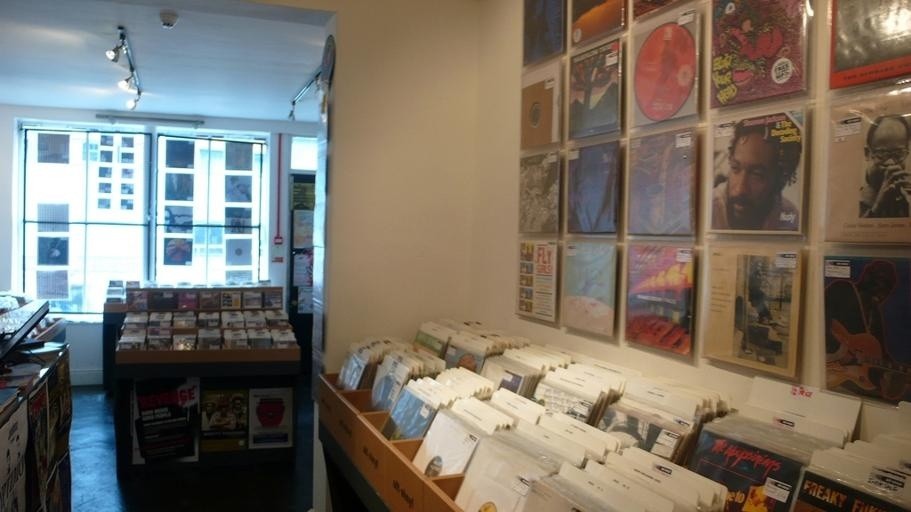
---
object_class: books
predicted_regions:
[480,343,638,424]
[455,412,620,512]
[294,209,315,250]
[520,447,726,510]
[791,434,911,510]
[296,285,314,314]
[333,338,416,389]
[128,375,200,465]
[366,349,446,409]
[292,182,316,211]
[688,402,848,510]
[293,254,313,287]
[597,373,732,464]
[413,317,531,374]
[383,366,498,440]
[411,386,550,477]
[117,288,297,348]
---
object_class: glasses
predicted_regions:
[867,142,909,162]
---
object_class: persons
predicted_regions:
[825,260,899,366]
[858,114,911,218]
[165,241,176,264]
[712,113,800,230]
[749,261,774,323]
[209,397,247,427]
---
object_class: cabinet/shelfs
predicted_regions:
[114,285,302,481]
[317,317,818,512]
